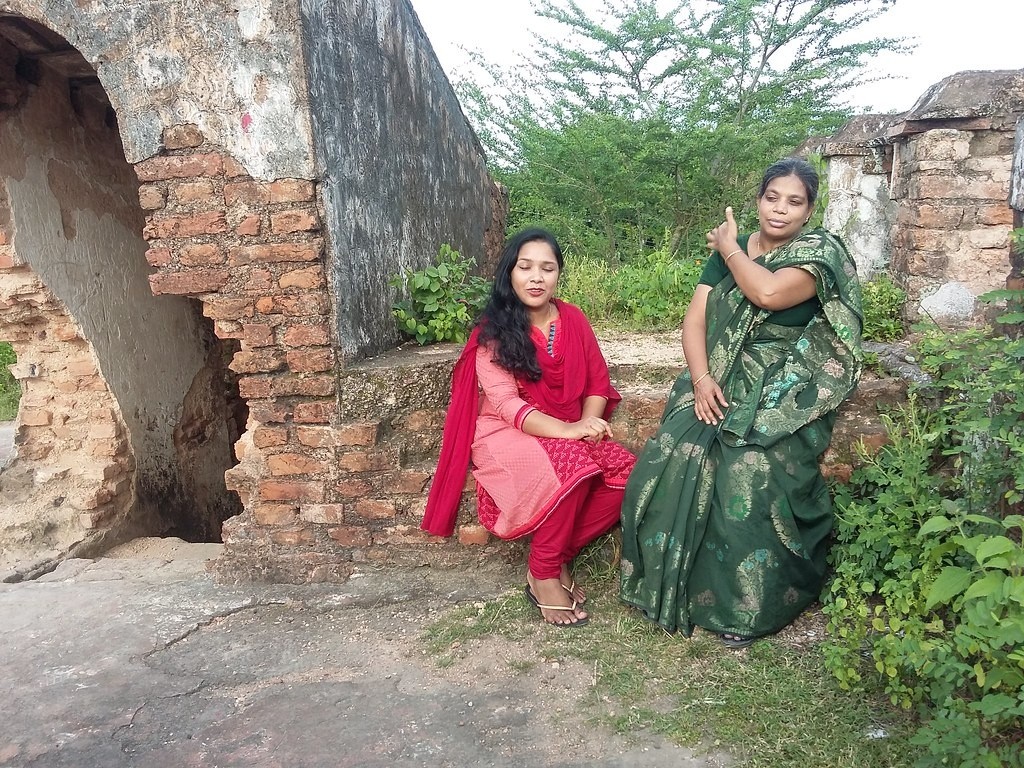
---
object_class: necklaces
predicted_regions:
[531,299,552,329]
[756,228,764,259]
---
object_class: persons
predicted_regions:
[465,226,636,627]
[619,160,865,650]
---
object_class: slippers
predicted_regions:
[525,578,590,627]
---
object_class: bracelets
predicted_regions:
[692,370,711,385]
[724,250,744,263]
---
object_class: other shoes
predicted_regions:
[720,634,756,649]
